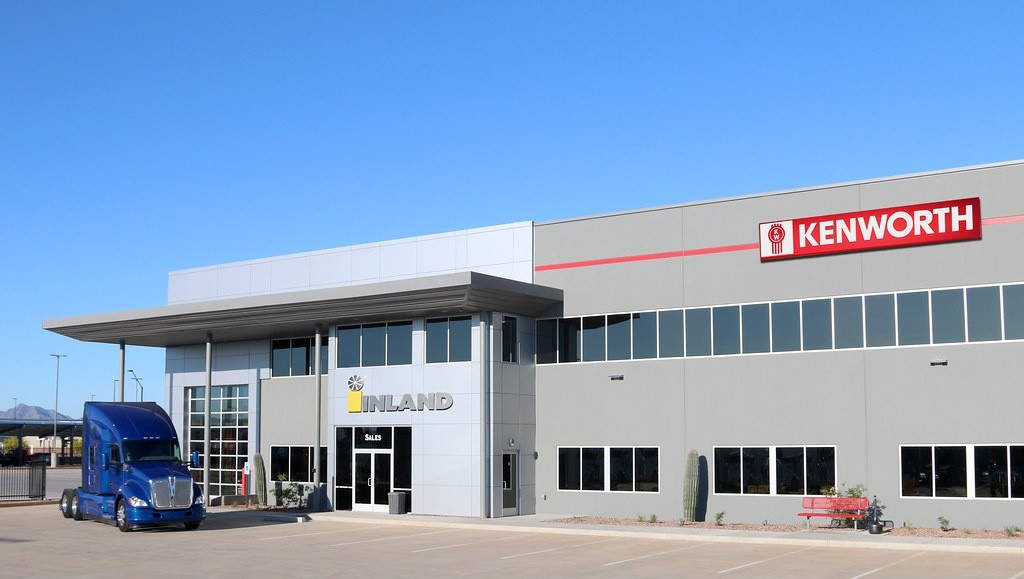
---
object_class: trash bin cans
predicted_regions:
[387,492,406,514]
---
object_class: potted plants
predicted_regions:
[822,483,867,529]
[868,499,886,534]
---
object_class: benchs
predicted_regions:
[797,497,869,532]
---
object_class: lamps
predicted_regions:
[608,374,624,381]
[930,359,948,365]
[509,438,515,446]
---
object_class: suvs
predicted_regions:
[3,449,28,467]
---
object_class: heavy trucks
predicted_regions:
[58,400,207,533]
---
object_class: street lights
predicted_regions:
[113,379,119,402]
[12,397,17,420]
[92,395,96,401]
[127,369,144,403]
[49,353,68,469]
[131,377,143,403]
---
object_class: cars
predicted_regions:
[31,453,51,465]
